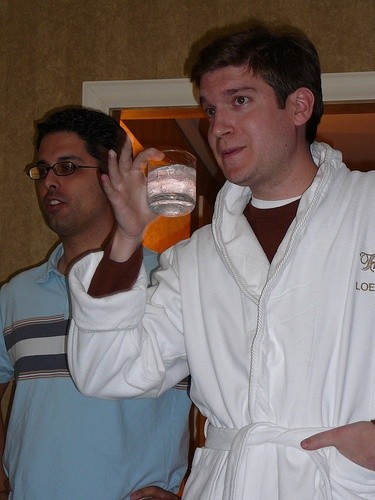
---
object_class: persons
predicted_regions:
[67,15,375,500]
[0,106,193,500]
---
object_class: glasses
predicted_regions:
[23,161,100,180]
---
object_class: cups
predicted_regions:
[146,149,197,218]
